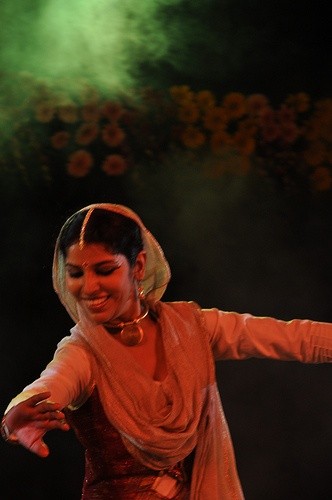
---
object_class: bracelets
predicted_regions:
[0,416,18,445]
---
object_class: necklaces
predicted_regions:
[102,299,150,346]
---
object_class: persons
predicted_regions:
[0,202,332,500]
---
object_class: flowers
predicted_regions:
[24,85,332,192]
